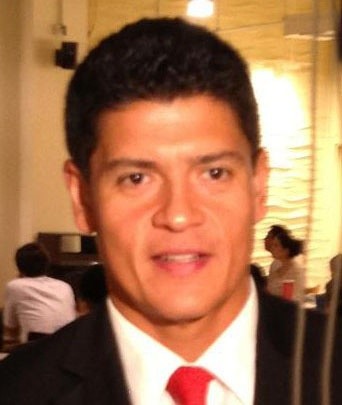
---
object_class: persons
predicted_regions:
[263,221,308,303]
[0,16,342,405]
[3,242,79,344]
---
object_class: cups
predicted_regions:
[280,279,294,300]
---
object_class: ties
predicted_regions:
[165,367,216,405]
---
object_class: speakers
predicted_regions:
[60,42,77,68]
